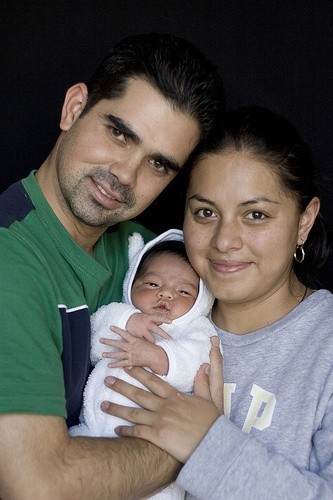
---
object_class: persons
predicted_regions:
[97,103,332,499]
[0,32,224,499]
[66,226,224,500]
